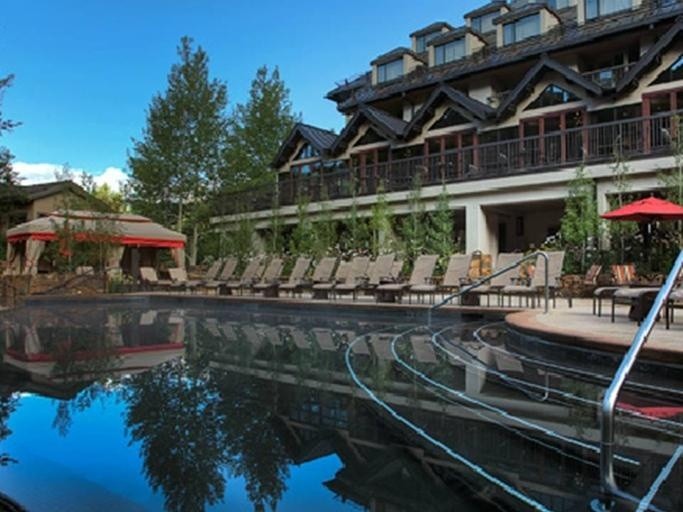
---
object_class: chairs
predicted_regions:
[562,264,683,331]
[410,253,472,306]
[502,252,566,309]
[76,252,439,304]
[462,253,523,307]
[140,309,557,381]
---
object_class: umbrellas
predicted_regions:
[599,192,683,270]
